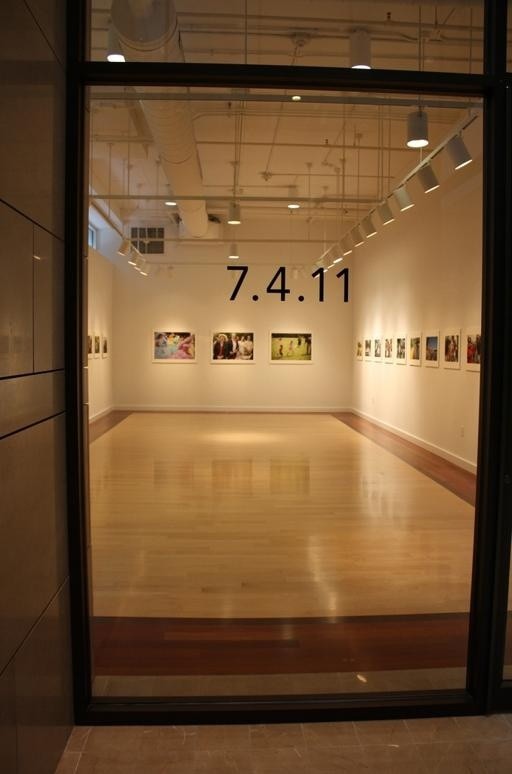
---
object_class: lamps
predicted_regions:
[226,200,243,259]
[347,32,371,69]
[316,100,473,274]
[118,237,151,278]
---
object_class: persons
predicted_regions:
[385,334,480,363]
[155,332,193,359]
[277,334,311,356]
[213,333,253,360]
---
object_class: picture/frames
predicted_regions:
[150,329,198,364]
[355,328,481,372]
[209,330,255,364]
[88,333,108,360]
[267,330,313,364]
